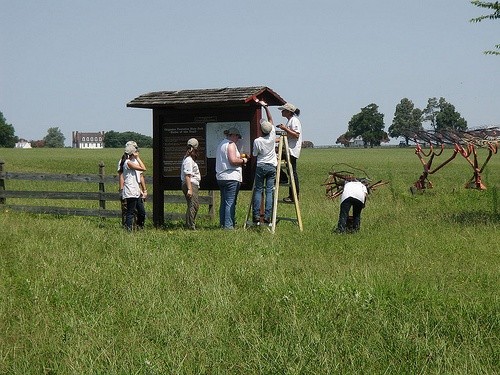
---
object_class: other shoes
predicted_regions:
[254,219,260,222]
[283,196,299,203]
[263,217,270,223]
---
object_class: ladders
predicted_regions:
[242,128,304,233]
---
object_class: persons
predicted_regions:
[334,182,368,233]
[118,141,147,231]
[216,128,251,228]
[252,101,277,226]
[181,138,201,230]
[278,103,302,202]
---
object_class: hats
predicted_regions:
[278,103,296,113]
[259,118,272,134]
[125,141,138,156]
[227,127,242,139]
[187,138,198,149]
